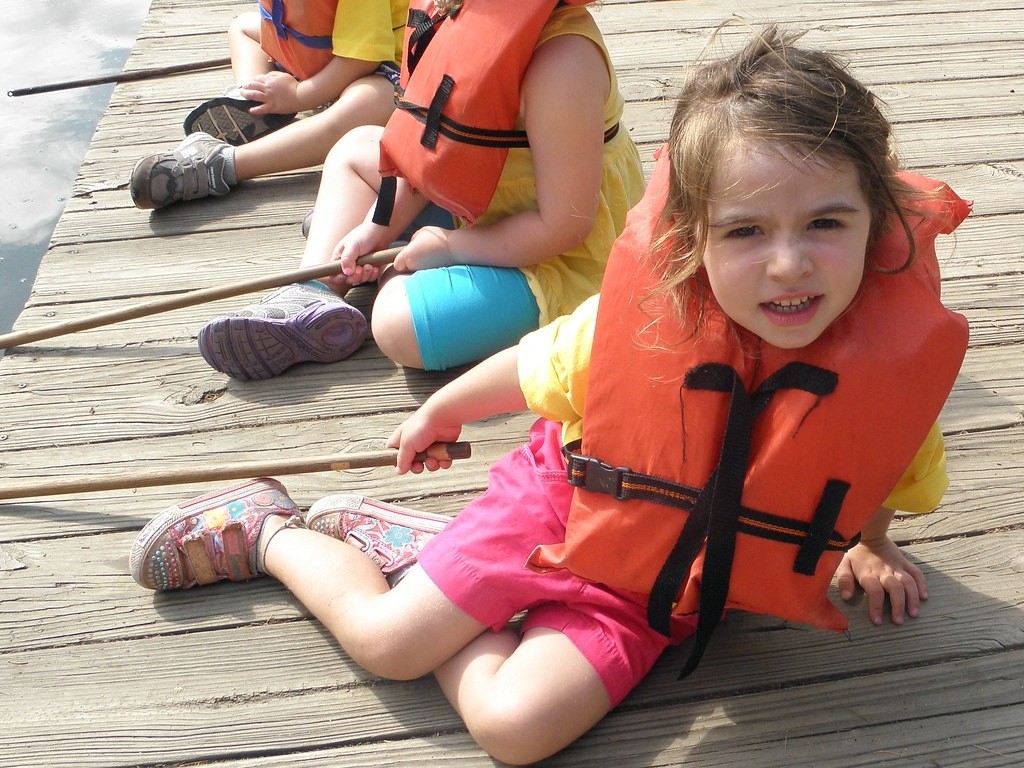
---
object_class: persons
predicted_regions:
[128,0,408,212]
[196,1,645,382]
[128,44,975,765]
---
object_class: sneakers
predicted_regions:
[128,479,305,590]
[196,282,369,381]
[183,82,298,147]
[305,493,459,578]
[129,132,235,209]
[302,208,421,242]
